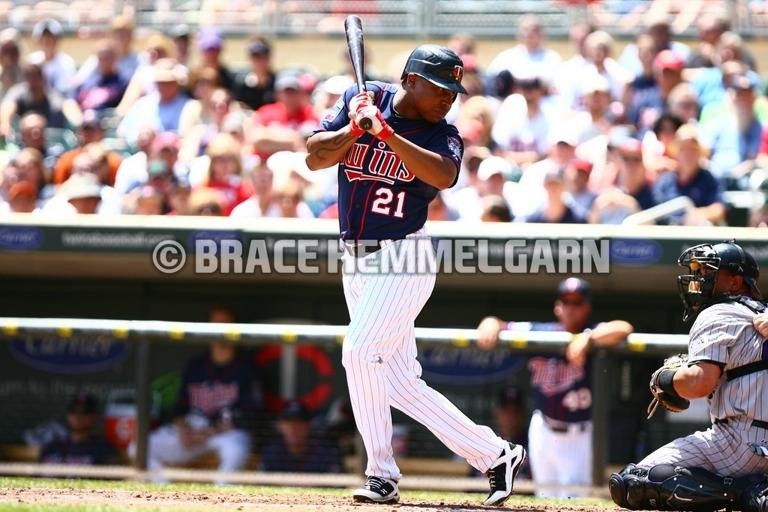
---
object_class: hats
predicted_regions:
[556,277,592,305]
[63,390,99,406]
[1,27,757,207]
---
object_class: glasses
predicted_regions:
[66,409,98,416]
[559,296,587,306]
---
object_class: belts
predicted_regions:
[715,413,767,430]
[539,412,592,431]
[337,236,399,257]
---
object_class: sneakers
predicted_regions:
[353,476,400,504]
[482,440,528,504]
[746,474,767,511]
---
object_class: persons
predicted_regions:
[305,45,529,508]
[1,309,361,479]
[494,385,529,479]
[607,240,768,510]
[0,3,766,228]
[752,301,768,339]
[475,278,635,498]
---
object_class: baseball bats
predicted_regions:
[344,15,372,131]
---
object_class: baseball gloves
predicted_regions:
[648,353,690,420]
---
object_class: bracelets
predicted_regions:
[584,328,594,347]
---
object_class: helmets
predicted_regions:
[677,239,762,330]
